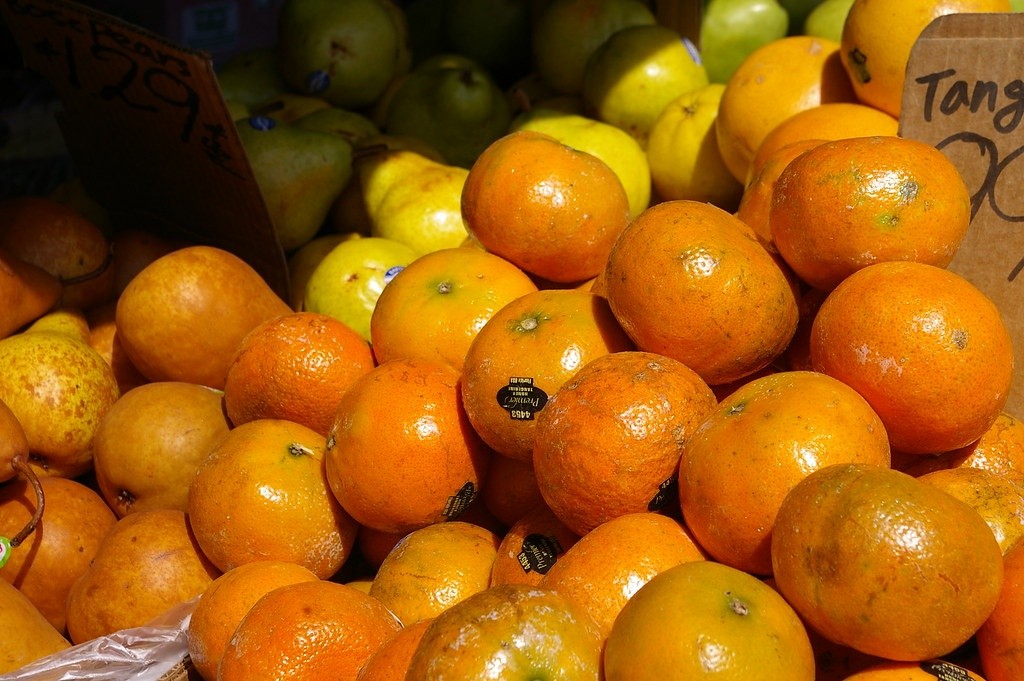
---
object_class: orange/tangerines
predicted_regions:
[0,0,1024,681]
